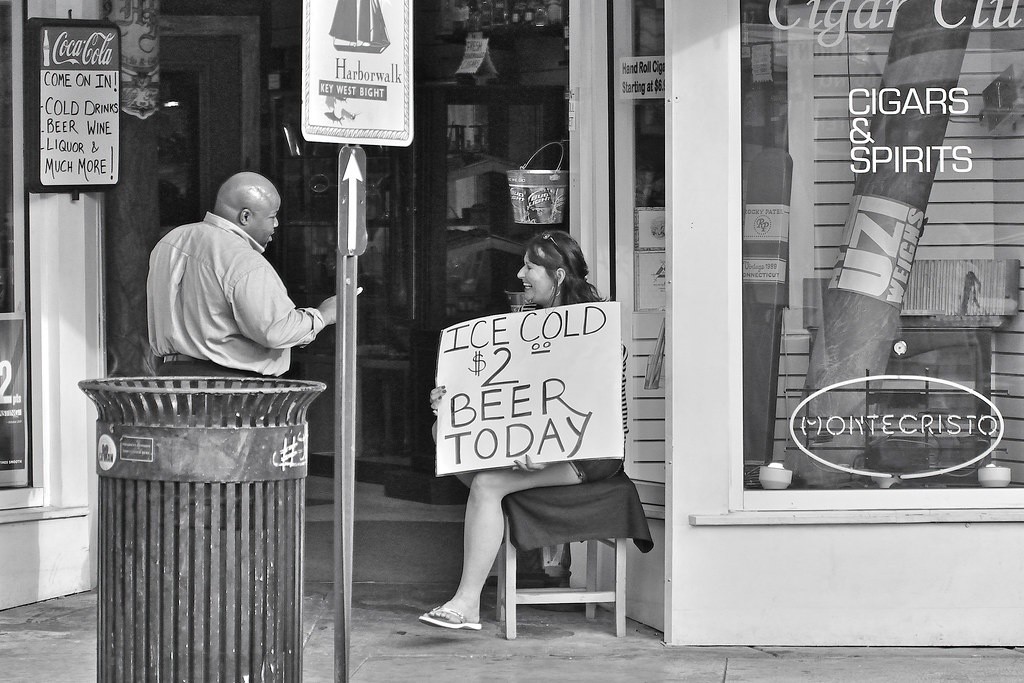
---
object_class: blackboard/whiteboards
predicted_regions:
[435,301,625,477]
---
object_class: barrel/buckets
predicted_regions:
[506,142,569,225]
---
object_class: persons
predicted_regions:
[418,230,628,630]
[146,171,364,379]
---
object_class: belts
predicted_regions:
[163,353,194,362]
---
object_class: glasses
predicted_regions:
[542,230,561,253]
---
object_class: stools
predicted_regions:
[495,487,656,637]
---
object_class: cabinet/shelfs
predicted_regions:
[268,80,570,468]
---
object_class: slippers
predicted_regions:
[418,606,482,631]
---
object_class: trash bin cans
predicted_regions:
[75,377,329,683]
